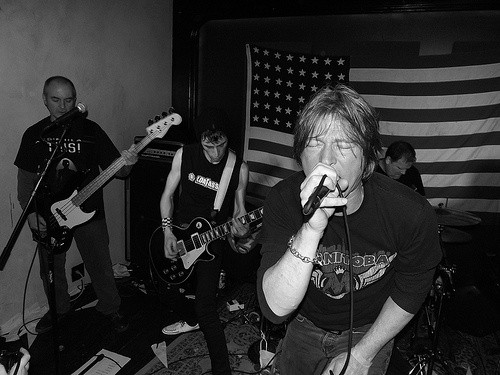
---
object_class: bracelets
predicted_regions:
[287,235,318,264]
[162,217,173,232]
[351,347,373,368]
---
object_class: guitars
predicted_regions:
[35,106,184,249]
[150,206,265,285]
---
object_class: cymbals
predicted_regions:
[435,206,482,226]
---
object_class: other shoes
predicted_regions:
[35,310,66,333]
[105,310,129,331]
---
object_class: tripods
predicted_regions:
[406,285,454,375]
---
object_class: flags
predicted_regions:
[242,43,500,225]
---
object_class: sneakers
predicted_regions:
[162,321,200,336]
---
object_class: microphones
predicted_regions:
[304,174,330,216]
[46,102,87,129]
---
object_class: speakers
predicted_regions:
[127,155,182,261]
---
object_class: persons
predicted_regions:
[257,84,443,375]
[13,76,139,333]
[374,141,425,197]
[160,112,251,335]
